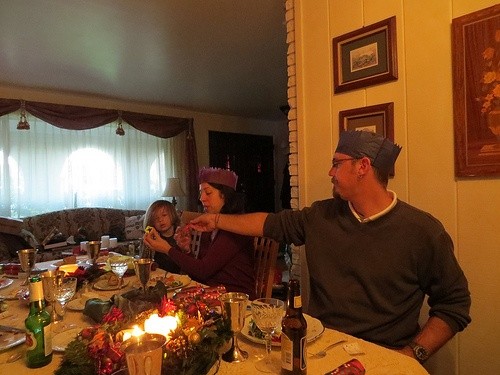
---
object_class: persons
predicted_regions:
[143,167,257,302]
[143,200,181,275]
[191,129,472,365]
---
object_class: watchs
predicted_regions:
[407,340,430,365]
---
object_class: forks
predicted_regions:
[309,340,348,359]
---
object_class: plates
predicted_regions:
[50,255,137,276]
[94,279,130,290]
[52,328,92,352]
[66,296,107,310]
[0,275,27,352]
[241,312,324,346]
[133,275,190,291]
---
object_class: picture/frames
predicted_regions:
[334,16,400,96]
[451,4,500,177]
[338,102,395,178]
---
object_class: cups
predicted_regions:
[81,234,117,255]
[120,333,167,375]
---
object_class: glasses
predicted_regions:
[332,157,356,167]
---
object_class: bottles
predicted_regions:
[24,276,52,368]
[280,277,307,375]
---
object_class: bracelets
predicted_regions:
[215,214,221,229]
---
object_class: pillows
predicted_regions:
[125,215,146,240]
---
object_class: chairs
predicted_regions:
[181,211,202,256]
[252,235,281,301]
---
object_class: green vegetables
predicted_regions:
[164,279,183,288]
[248,318,266,340]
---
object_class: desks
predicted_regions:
[0,250,427,375]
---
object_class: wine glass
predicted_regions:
[38,270,78,333]
[16,248,38,285]
[87,240,102,268]
[218,292,285,373]
[132,259,154,294]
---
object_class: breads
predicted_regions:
[108,274,124,286]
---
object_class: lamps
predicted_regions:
[162,177,187,207]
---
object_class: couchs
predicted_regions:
[20,206,147,264]
